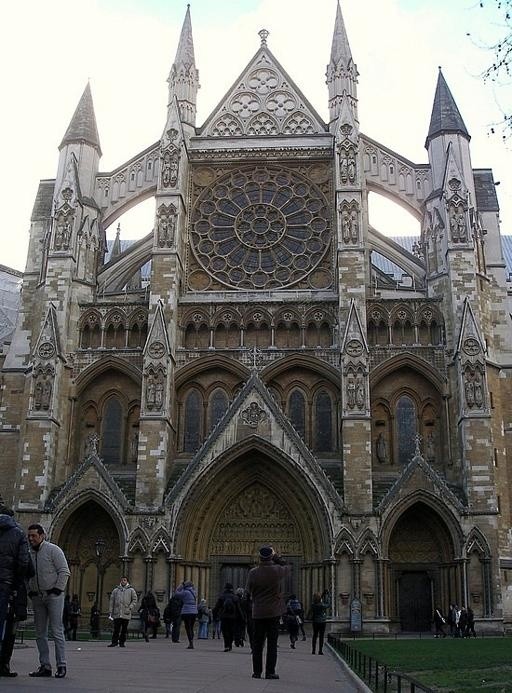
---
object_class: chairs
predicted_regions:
[223,642,244,652]
[165,636,169,638]
[318,652,324,655]
[1,672,17,677]
[295,638,298,641]
[218,637,220,639]
[146,639,149,641]
[213,637,215,639]
[108,642,118,647]
[312,652,316,655]
[252,673,264,678]
[265,674,279,679]
[300,637,306,641]
[187,645,194,649]
[172,641,180,643]
[149,636,157,639]
[290,644,296,649]
[119,643,125,647]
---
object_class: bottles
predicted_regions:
[260,547,272,558]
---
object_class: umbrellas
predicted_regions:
[29,667,52,677]
[55,666,66,678]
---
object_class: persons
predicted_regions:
[3,551,36,677]
[0,502,30,677]
[28,523,73,678]
[241,544,293,679]
